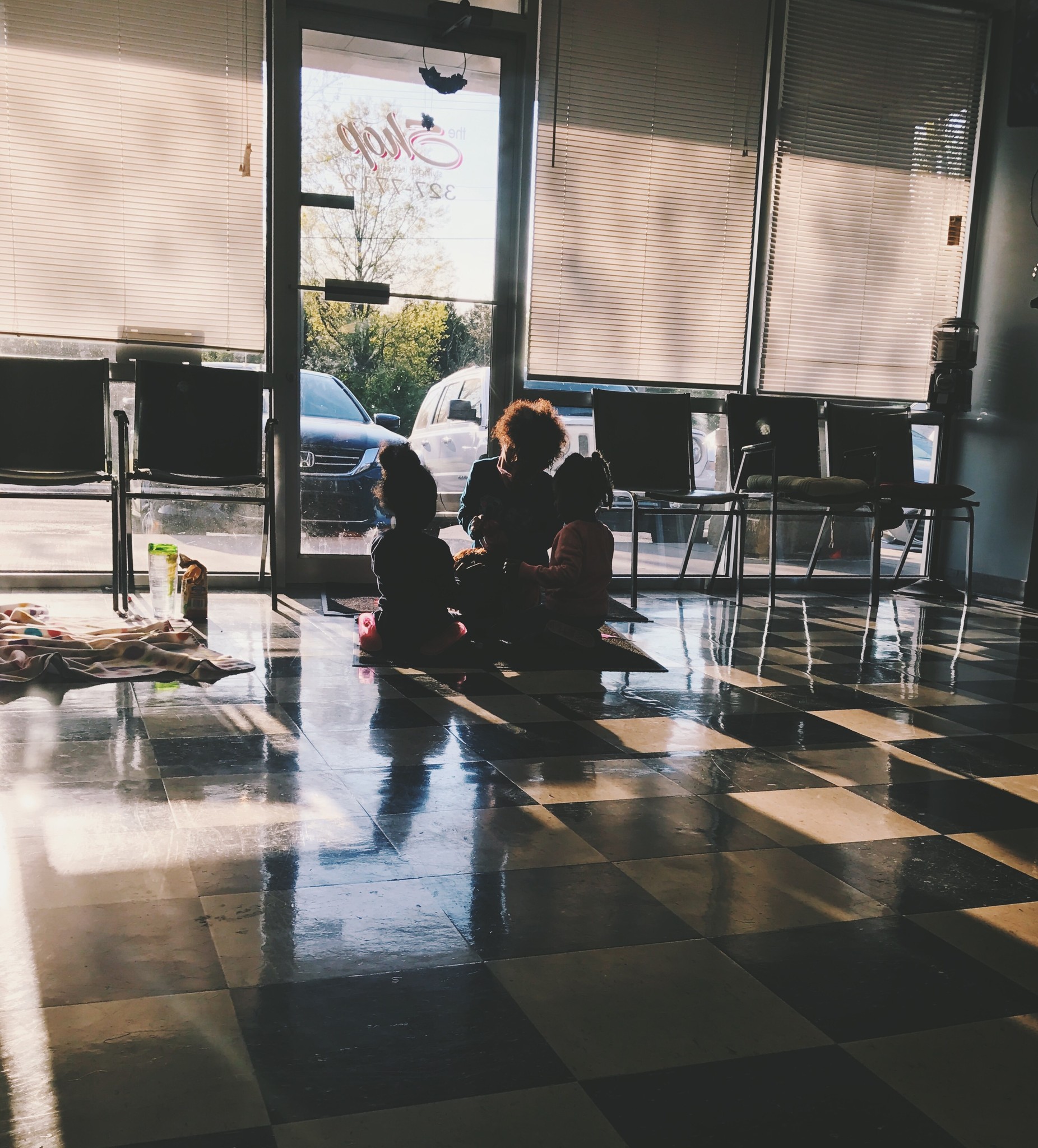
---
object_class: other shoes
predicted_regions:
[547,619,595,647]
[359,612,382,652]
[420,621,467,655]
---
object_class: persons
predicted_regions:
[360,443,467,655]
[460,396,567,569]
[502,451,616,645]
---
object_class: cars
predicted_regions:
[202,361,409,536]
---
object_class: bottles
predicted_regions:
[148,543,178,620]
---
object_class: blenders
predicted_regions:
[925,317,979,414]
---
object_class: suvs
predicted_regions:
[406,365,640,527]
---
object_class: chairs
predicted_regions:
[817,400,979,606]
[115,358,280,612]
[583,392,750,609]
[723,392,883,606]
[0,353,122,612]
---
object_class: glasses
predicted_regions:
[496,450,543,485]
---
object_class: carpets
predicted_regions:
[321,580,648,623]
[352,610,669,673]
